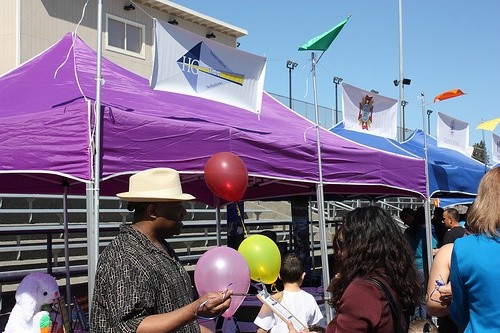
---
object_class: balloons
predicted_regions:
[195,247,251,320]
[240,233,281,286]
[203,150,250,204]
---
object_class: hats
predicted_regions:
[116,167,196,202]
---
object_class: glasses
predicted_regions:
[337,238,344,251]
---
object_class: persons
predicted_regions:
[253,252,324,333]
[400,202,476,333]
[437,165,500,333]
[89,167,233,333]
[287,206,429,333]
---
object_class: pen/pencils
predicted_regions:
[230,293,255,296]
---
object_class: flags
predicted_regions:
[297,17,348,52]
[476,118,500,132]
[434,89,464,103]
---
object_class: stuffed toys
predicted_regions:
[2,271,61,333]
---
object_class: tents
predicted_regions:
[0,31,494,324]
